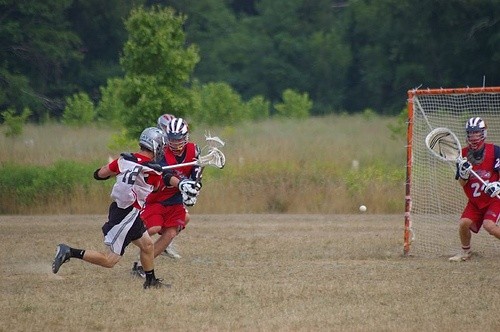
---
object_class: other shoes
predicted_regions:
[130,261,146,277]
[448,252,471,262]
[159,246,183,259]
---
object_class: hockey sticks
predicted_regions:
[425,127,500,201]
[194,136,225,186]
[161,149,226,170]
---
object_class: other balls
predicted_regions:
[360,205,367,211]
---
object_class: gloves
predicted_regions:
[178,178,201,208]
[483,181,500,197]
[459,159,473,180]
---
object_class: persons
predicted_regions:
[51,127,172,290]
[131,114,203,280]
[448,118,500,261]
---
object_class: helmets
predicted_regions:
[156,114,174,132]
[139,127,170,155]
[466,117,487,140]
[166,117,190,143]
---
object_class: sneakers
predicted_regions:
[143,278,171,290]
[52,243,73,275]
